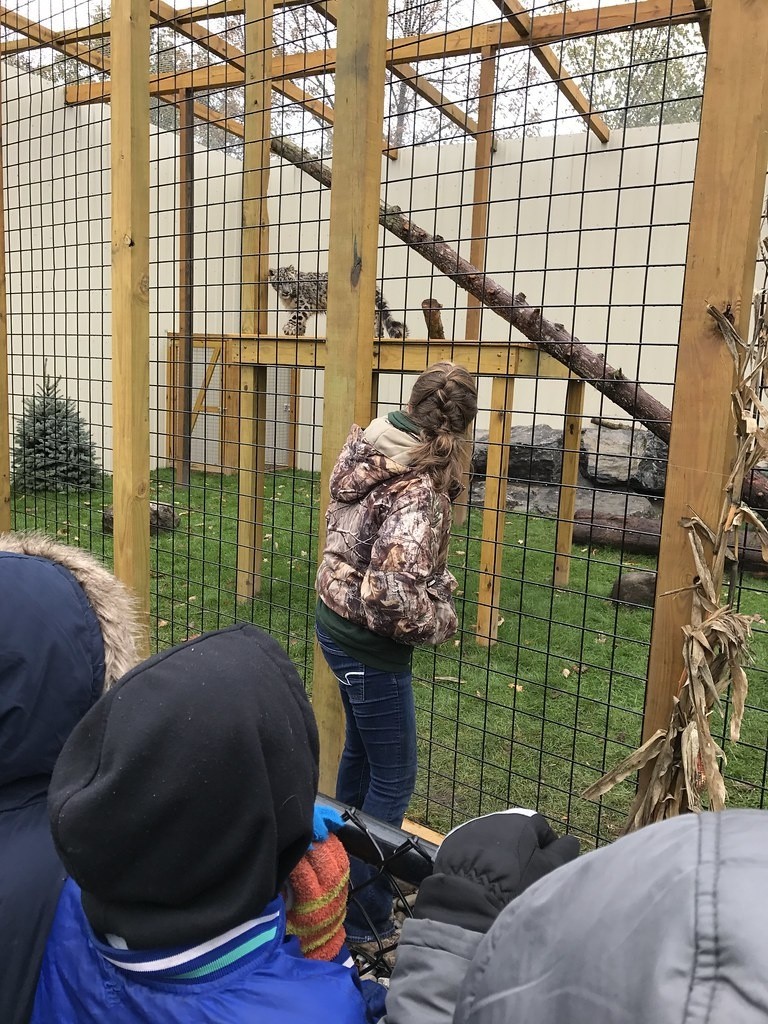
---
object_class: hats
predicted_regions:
[49,622,319,949]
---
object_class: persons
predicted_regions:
[29,624,388,1024]
[314,361,478,969]
[0,529,147,1024]
[376,807,768,1024]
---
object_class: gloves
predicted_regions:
[287,803,350,961]
[412,807,581,933]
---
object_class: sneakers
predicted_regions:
[346,932,399,969]
